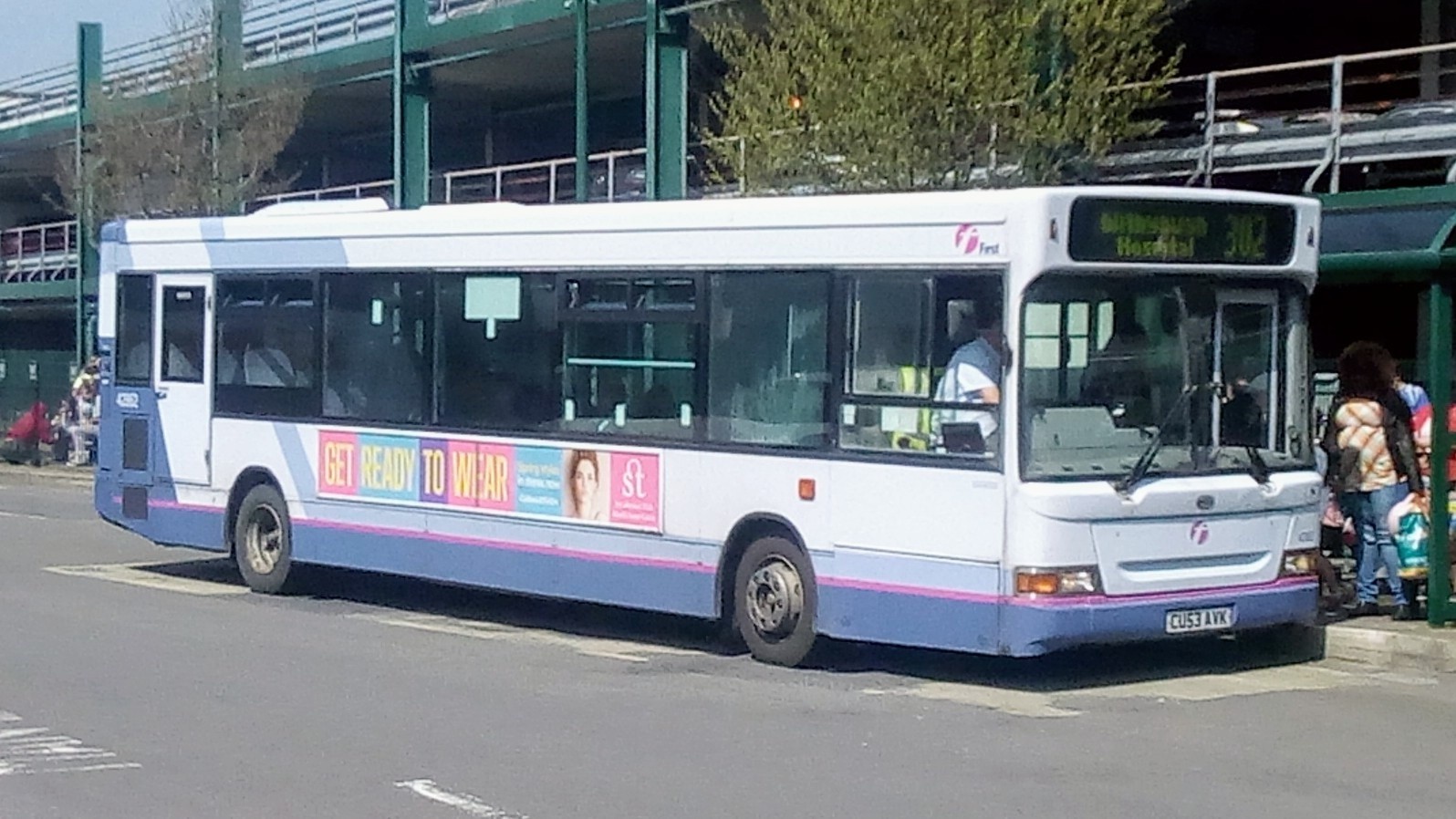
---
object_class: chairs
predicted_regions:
[880,364,942,451]
[126,320,321,386]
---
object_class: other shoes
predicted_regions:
[1346,602,1379,616]
[1392,604,1410,620]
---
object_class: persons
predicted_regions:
[1079,323,1148,407]
[1224,380,1264,447]
[931,311,1003,449]
[1314,342,1456,620]
[4,354,101,467]
[564,451,605,521]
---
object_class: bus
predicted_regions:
[89,182,1331,673]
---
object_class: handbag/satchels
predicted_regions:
[1389,492,1430,580]
